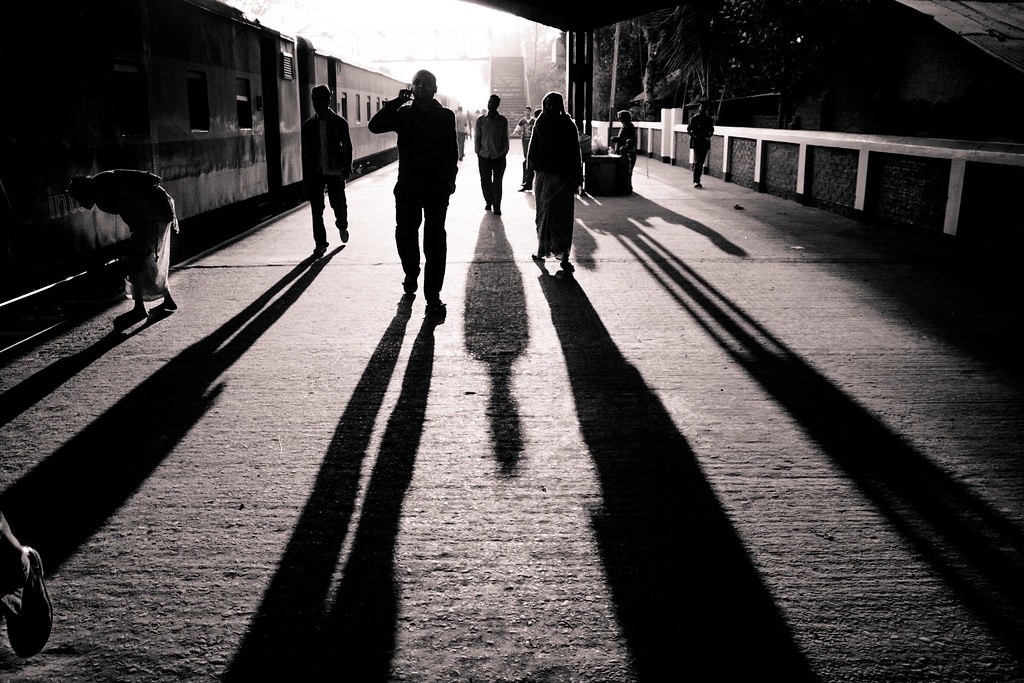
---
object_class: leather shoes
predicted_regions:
[5,545,53,658]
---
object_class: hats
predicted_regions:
[311,85,334,101]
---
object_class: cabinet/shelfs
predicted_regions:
[589,154,621,198]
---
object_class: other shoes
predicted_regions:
[693,182,702,188]
[314,247,327,255]
[560,261,575,272]
[532,252,546,262]
[340,229,349,243]
[485,204,501,215]
[518,182,532,192]
[402,267,421,294]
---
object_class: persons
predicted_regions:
[510,107,543,192]
[455,106,487,161]
[67,169,179,324]
[474,95,509,216]
[610,110,637,195]
[0,511,53,658]
[687,101,714,188]
[301,85,352,253]
[525,92,584,273]
[369,70,459,313]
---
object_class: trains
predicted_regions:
[1,1,453,344]
